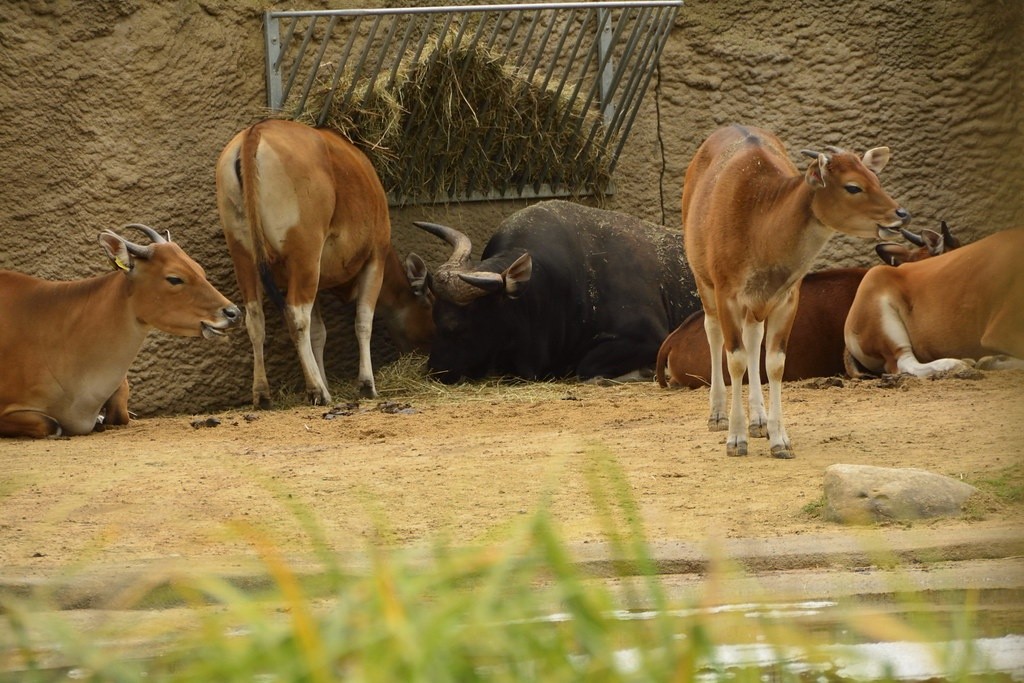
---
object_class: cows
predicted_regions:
[3,118,1023,460]
